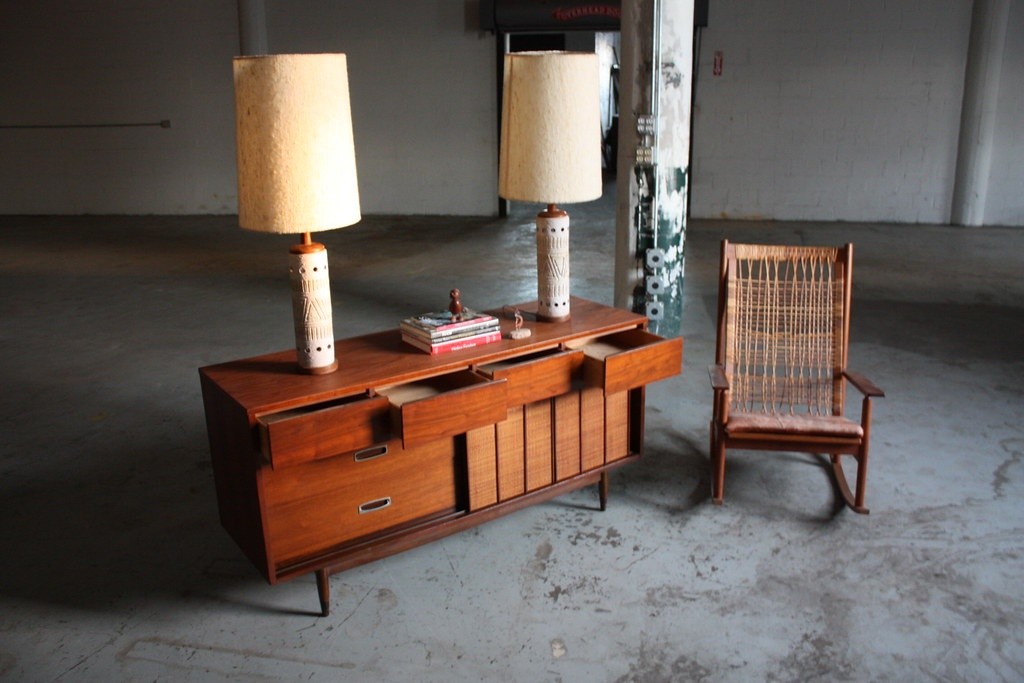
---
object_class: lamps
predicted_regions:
[230,53,361,377]
[494,50,602,323]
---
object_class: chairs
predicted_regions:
[709,240,884,518]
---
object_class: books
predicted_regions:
[400,306,502,356]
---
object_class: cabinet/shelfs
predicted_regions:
[196,295,683,615]
